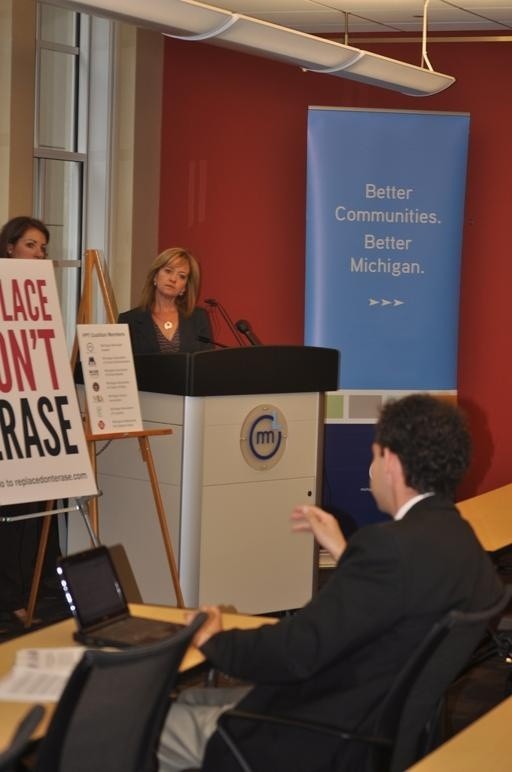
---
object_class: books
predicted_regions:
[0,646,92,707]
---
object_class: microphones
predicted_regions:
[198,334,228,348]
[203,299,247,346]
[235,320,263,346]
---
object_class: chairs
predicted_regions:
[205,586,512,772]
[1,612,207,772]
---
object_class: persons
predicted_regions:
[142,391,512,772]
[0,214,51,258]
[115,244,213,390]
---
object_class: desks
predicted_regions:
[453,483,512,552]
[406,696,512,772]
[0,601,283,755]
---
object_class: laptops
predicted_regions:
[54,545,188,649]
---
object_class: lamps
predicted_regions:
[49,0,456,96]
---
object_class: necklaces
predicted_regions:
[147,302,183,331]
